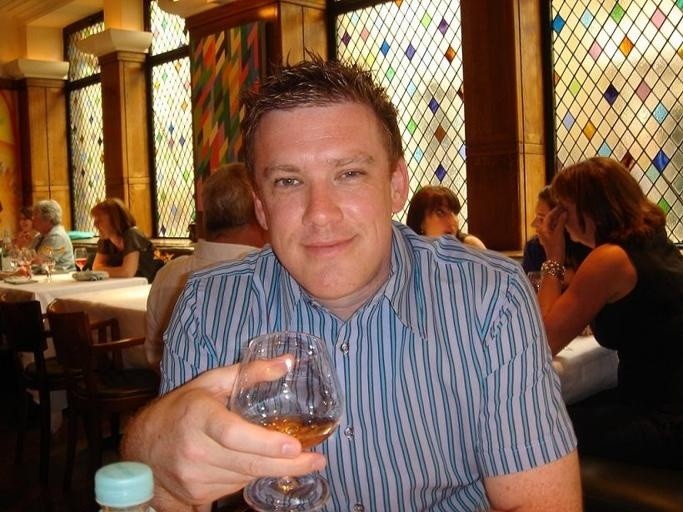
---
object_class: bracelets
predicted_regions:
[540,259,565,286]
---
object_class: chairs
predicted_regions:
[44,309,170,462]
[1,292,43,430]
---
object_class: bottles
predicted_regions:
[91,459,157,512]
[0,229,12,273]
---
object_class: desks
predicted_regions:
[546,320,624,431]
[9,259,185,310]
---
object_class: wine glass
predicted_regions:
[229,330,345,512]
[73,247,89,273]
[10,243,56,284]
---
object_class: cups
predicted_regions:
[526,271,544,294]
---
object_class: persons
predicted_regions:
[91,199,163,282]
[119,49,583,512]
[145,161,270,378]
[522,185,593,293]
[14,207,39,251]
[406,186,488,251]
[34,200,76,275]
[535,157,683,470]
